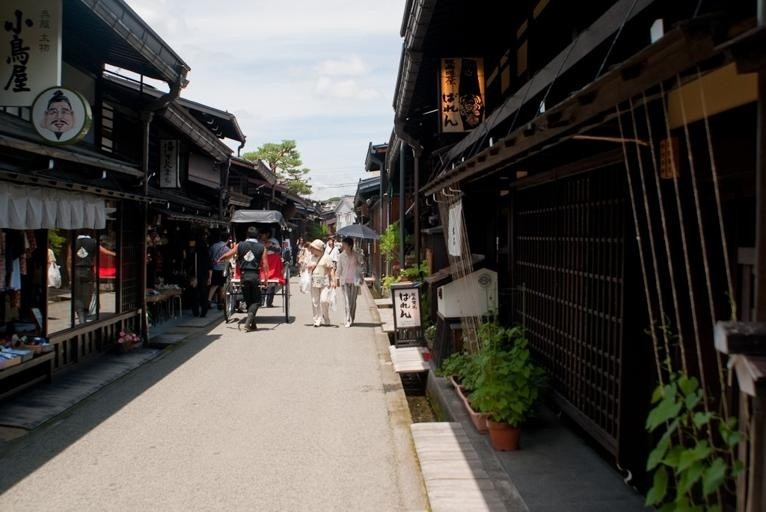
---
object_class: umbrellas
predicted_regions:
[337,223,380,246]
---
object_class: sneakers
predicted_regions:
[345,321,354,327]
[193,301,224,318]
[244,323,257,332]
[314,319,330,326]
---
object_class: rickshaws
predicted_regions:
[223,210,292,323]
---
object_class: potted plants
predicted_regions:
[424,325,437,350]
[433,296,552,453]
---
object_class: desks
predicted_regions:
[0,350,56,402]
[146,286,183,327]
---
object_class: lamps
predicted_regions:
[102,168,108,178]
[148,170,156,182]
[48,157,54,169]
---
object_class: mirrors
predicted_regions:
[73,228,99,326]
[98,216,120,322]
[43,224,74,337]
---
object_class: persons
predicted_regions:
[337,238,364,328]
[294,238,340,275]
[215,225,270,332]
[260,285,275,308]
[186,239,212,317]
[40,91,76,135]
[257,227,285,264]
[206,233,232,308]
[66,228,117,328]
[306,239,337,327]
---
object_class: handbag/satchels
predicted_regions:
[48,263,61,288]
[320,288,337,312]
[299,272,310,294]
[190,279,198,288]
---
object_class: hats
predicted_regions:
[306,239,325,252]
